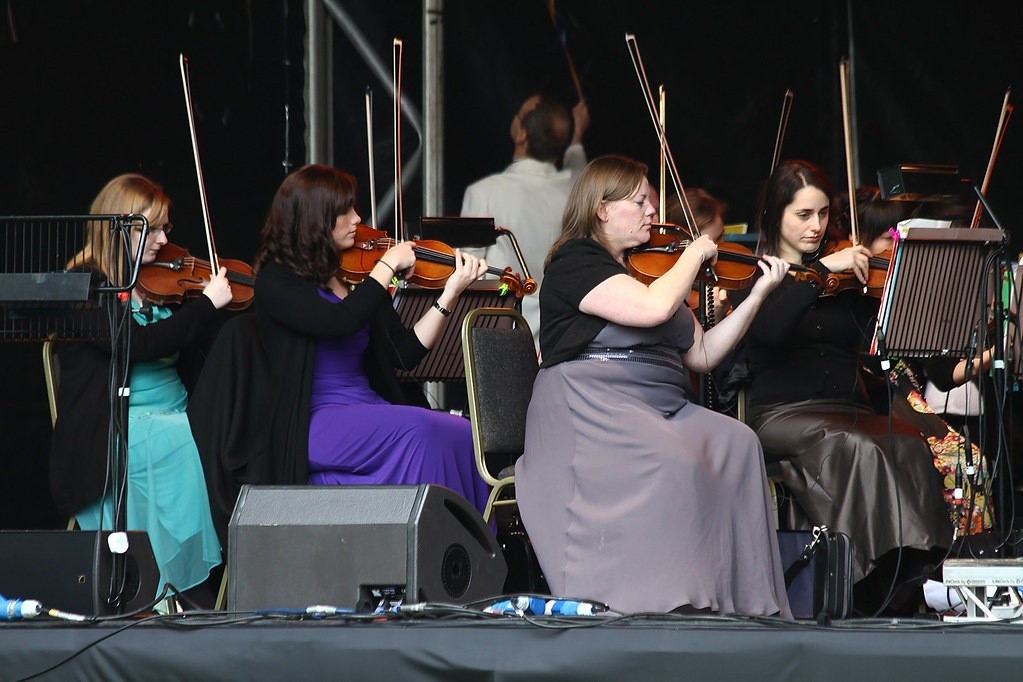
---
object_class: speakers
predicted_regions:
[0,529,161,620]
[227,485,510,609]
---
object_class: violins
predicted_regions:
[337,223,538,298]
[627,223,839,291]
[135,241,257,311]
[798,237,894,300]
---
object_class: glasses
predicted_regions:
[134,223,173,234]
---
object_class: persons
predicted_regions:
[42,171,233,599]
[653,185,726,392]
[254,163,498,538]
[661,187,991,251]
[514,153,796,622]
[458,89,591,370]
[719,159,955,617]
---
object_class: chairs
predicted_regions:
[738,389,787,531]
[461,308,540,522]
[43,332,80,531]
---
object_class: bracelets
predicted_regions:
[433,301,452,317]
[379,260,395,275]
